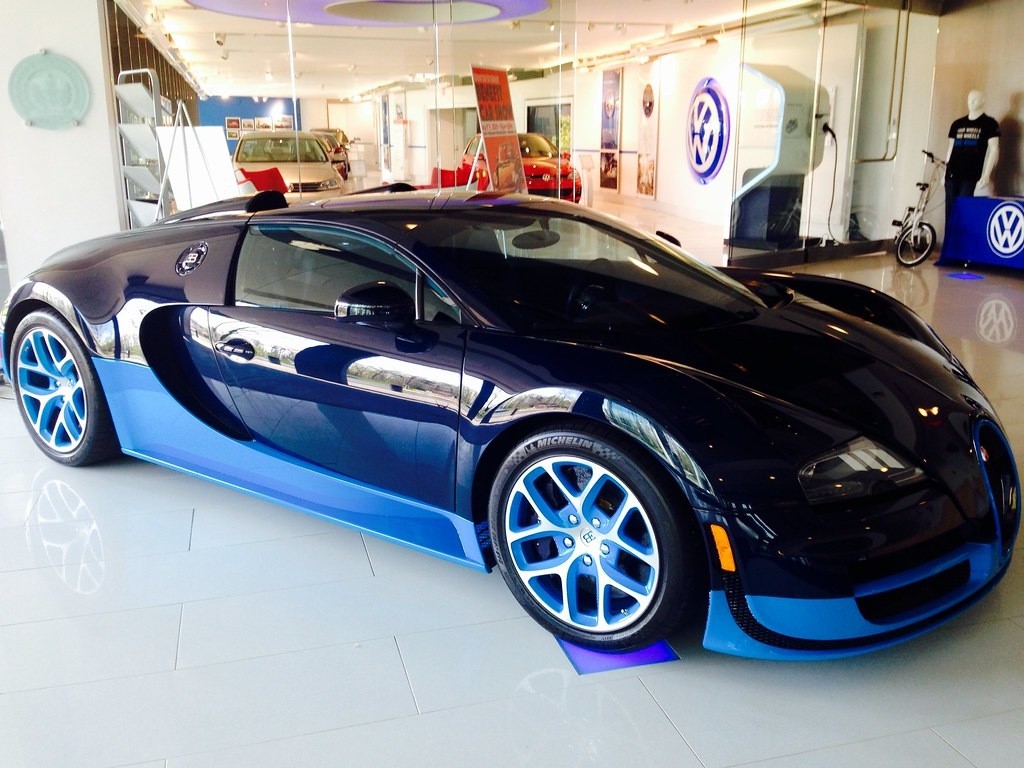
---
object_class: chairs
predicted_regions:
[288,145,314,161]
[244,145,270,161]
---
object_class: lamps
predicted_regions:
[214,33,226,45]
[221,51,230,59]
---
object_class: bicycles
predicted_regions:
[891,149,948,267]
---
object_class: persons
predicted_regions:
[941,88,1000,233]
[292,144,313,162]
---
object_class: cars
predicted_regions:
[231,131,345,195]
[310,132,349,181]
[458,132,582,202]
[324,133,349,175]
[310,128,349,156]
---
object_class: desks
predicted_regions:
[933,195,1024,270]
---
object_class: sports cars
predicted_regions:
[0,190,1023,662]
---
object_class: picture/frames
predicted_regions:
[225,115,293,140]
